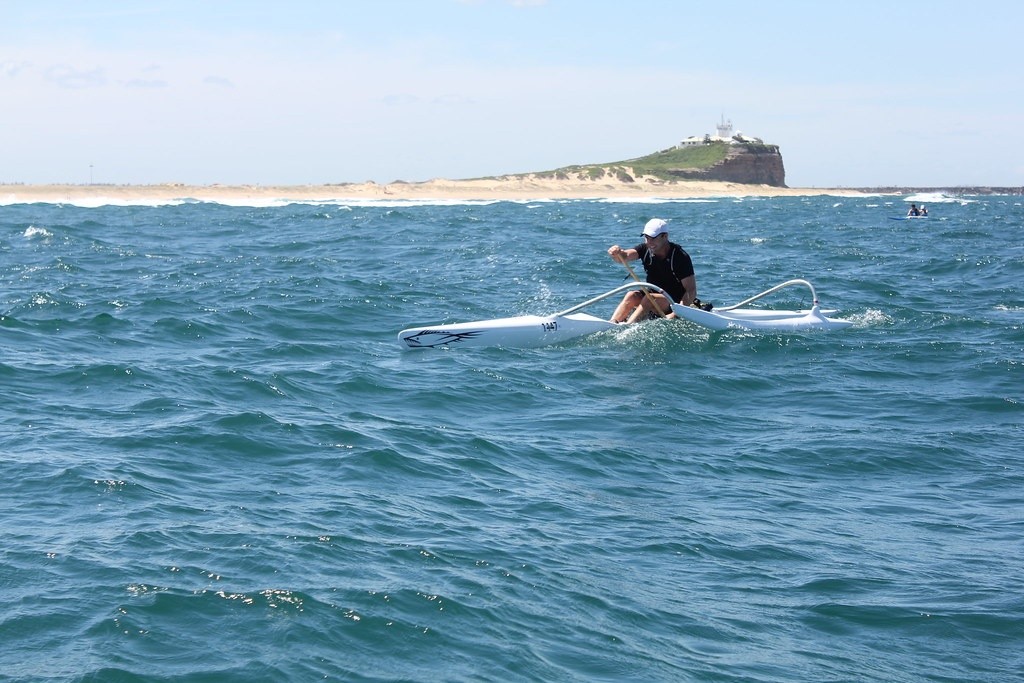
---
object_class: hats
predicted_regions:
[640,218,668,239]
[920,205,925,210]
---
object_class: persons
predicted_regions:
[906,203,929,216]
[606,217,697,325]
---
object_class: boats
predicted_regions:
[886,215,950,221]
[397,273,855,352]
